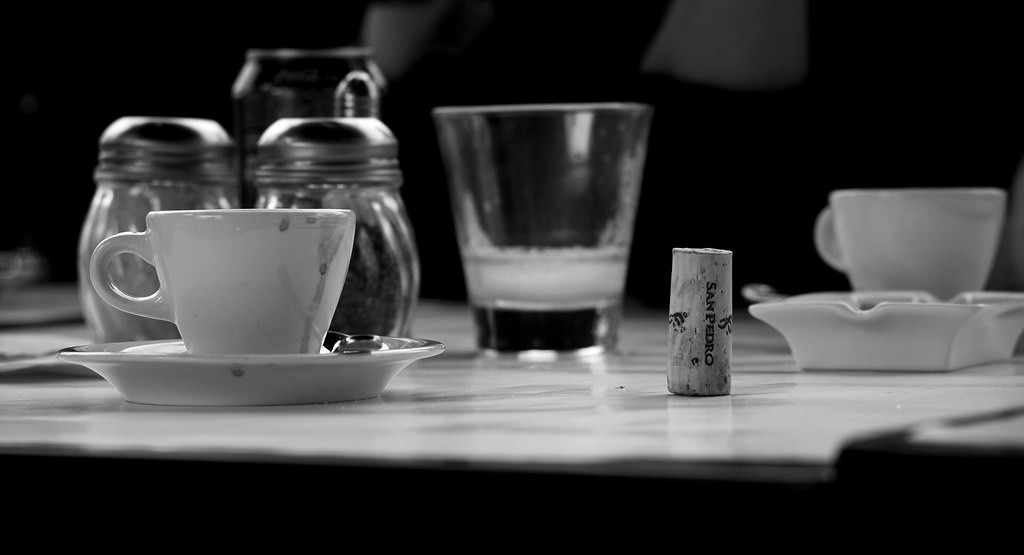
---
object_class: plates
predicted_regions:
[56,331,447,410]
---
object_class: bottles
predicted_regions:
[75,113,421,344]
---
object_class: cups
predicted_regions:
[86,207,356,355]
[813,187,1006,292]
[432,102,654,362]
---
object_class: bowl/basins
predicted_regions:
[748,293,1024,372]
